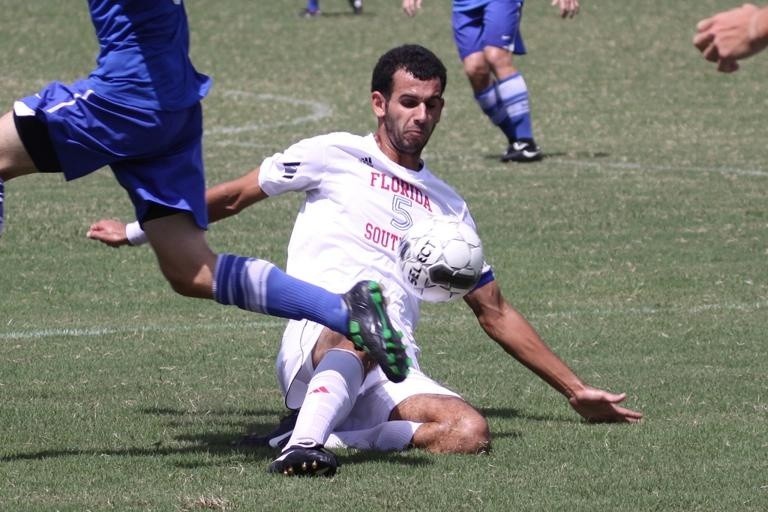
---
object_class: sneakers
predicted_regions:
[501,141,542,163]
[269,439,338,478]
[269,413,295,448]
[343,280,411,383]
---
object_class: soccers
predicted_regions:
[398,217,481,301]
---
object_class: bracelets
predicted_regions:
[125,219,147,247]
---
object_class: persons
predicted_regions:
[299,0,361,18]
[84,42,642,478]
[691,0,767,73]
[0,0,413,383]
[400,0,579,165]
